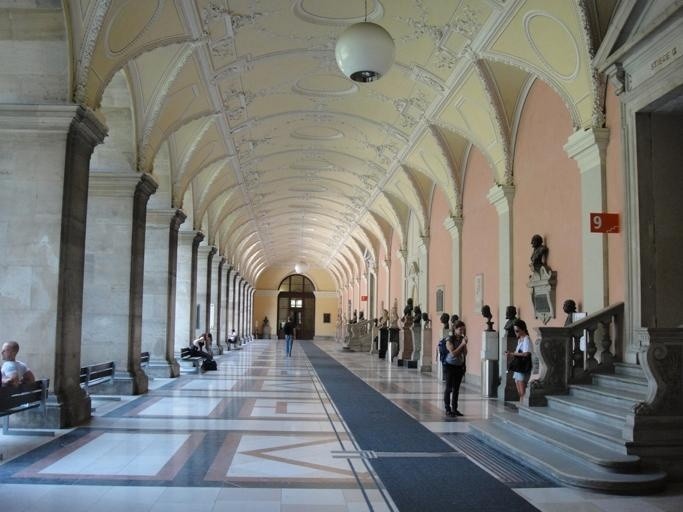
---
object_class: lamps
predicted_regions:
[333,0,397,83]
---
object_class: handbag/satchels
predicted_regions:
[507,352,531,373]
[200,359,217,370]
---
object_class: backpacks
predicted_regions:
[437,336,447,362]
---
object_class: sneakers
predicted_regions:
[444,409,463,417]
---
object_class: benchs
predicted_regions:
[0,345,202,462]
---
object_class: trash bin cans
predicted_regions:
[264,326,270,338]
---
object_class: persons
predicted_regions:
[530,234,545,270]
[563,299,576,326]
[349,310,364,324]
[404,298,431,325]
[0,341,35,386]
[440,313,458,329]
[438,321,468,417]
[504,306,521,337]
[191,333,213,361]
[481,305,496,331]
[505,320,532,403]
[284,317,296,357]
[263,316,269,324]
[228,329,236,343]
